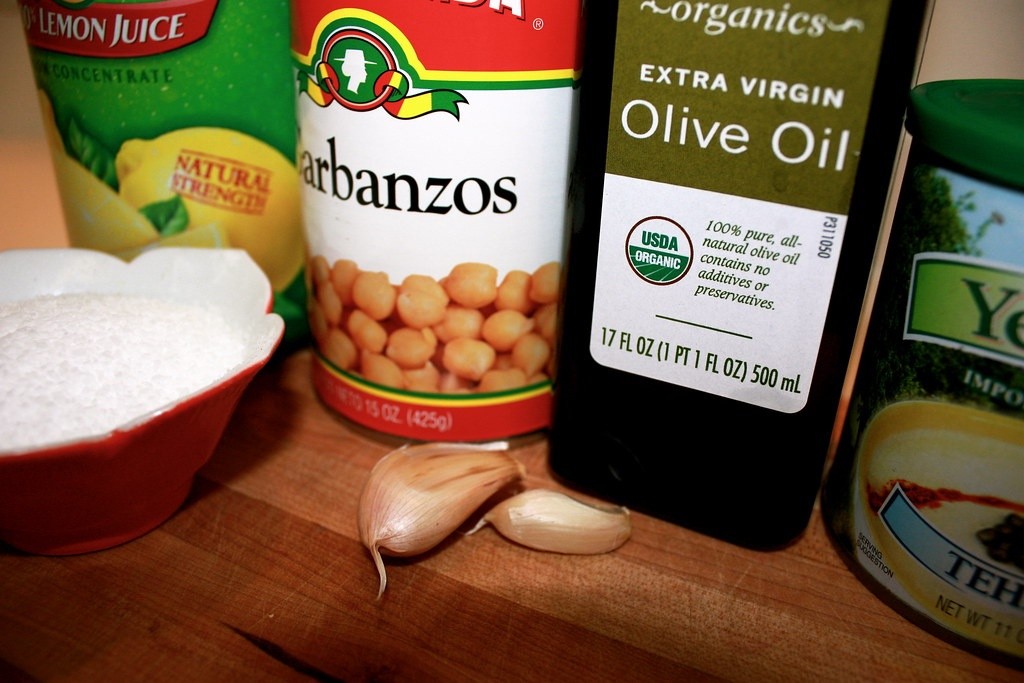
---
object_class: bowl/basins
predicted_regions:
[0,245,285,559]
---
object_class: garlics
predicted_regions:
[358,441,633,600]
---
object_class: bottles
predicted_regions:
[820,78,1024,670]
[291,0,580,449]
[14,0,306,355]
[545,1,937,551]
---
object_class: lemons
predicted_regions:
[50,126,305,296]
